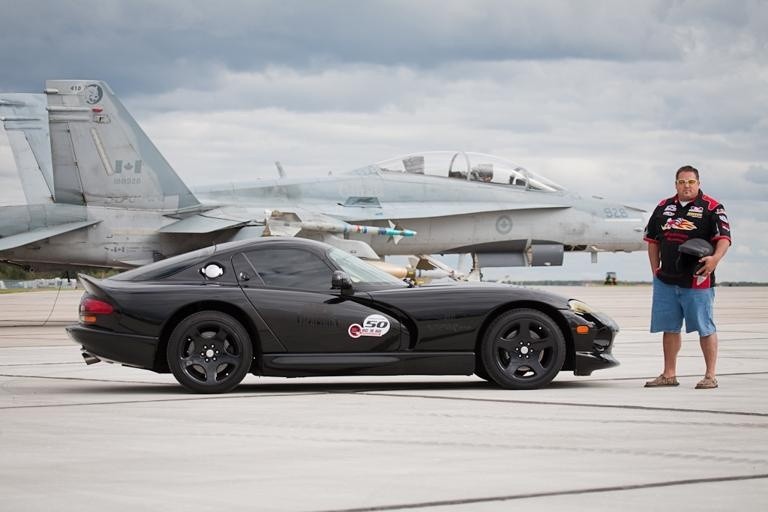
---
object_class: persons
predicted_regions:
[642,166,732,389]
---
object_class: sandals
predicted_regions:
[695,373,719,389]
[644,373,680,387]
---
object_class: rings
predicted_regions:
[709,271,712,274]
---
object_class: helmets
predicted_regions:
[674,238,715,276]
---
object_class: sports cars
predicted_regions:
[64,238,620,393]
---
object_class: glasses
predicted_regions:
[674,179,699,187]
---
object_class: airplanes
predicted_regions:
[0,78,649,286]
